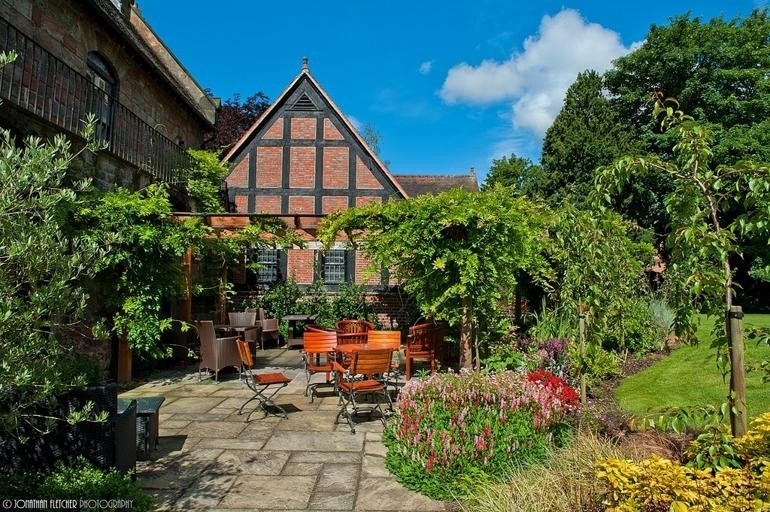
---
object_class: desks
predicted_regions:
[117,396,165,461]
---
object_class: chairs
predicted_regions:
[0,383,137,483]
[191,306,449,435]
